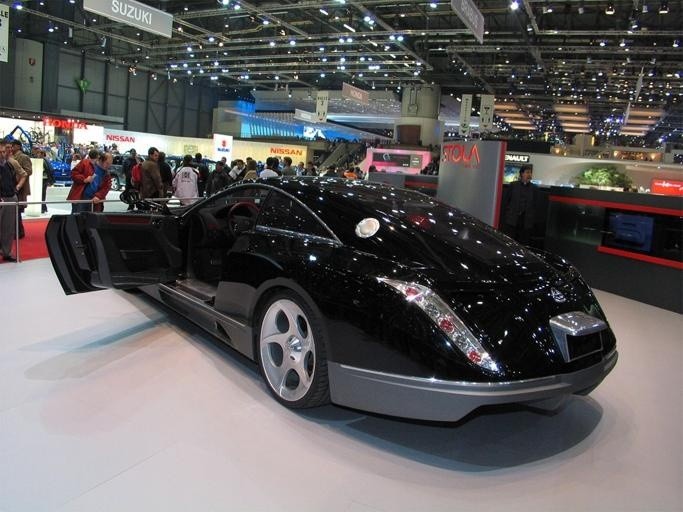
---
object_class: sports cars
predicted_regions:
[44,176,618,422]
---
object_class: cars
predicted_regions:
[5,126,74,186]
[109,151,216,189]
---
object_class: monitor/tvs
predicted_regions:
[601,208,657,257]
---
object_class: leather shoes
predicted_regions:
[2,255,16,262]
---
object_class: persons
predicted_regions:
[324,137,364,180]
[420,158,439,176]
[500,163,538,248]
[0,138,19,265]
[10,139,32,240]
[67,140,118,213]
[0,138,28,256]
[205,156,318,197]
[31,142,58,213]
[121,147,207,210]
